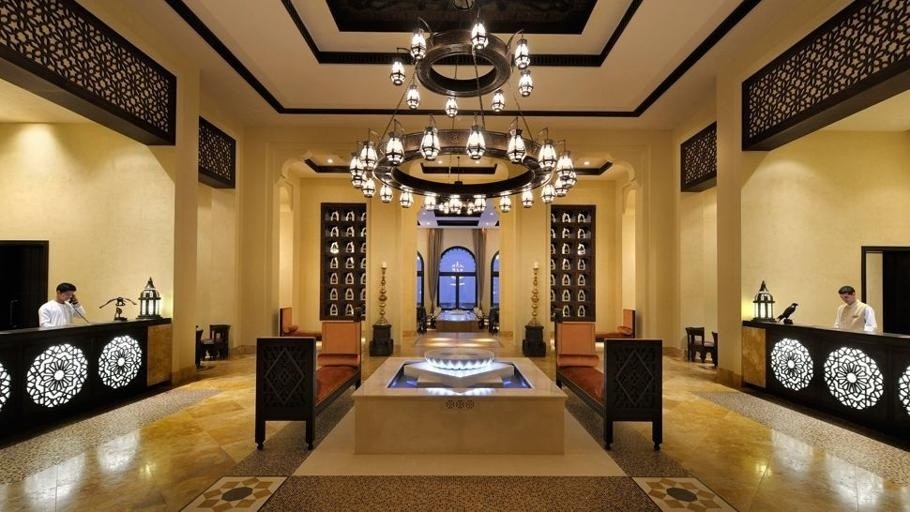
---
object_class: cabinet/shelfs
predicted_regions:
[320,203,366,321]
[550,204,597,323]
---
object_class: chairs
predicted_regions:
[686,327,714,363]
[202,324,231,369]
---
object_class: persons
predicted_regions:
[833,286,877,333]
[38,283,86,329]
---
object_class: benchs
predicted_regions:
[552,316,662,454]
[255,320,362,450]
[280,307,322,341]
[596,308,635,341]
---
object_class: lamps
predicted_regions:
[752,280,775,322]
[350,0,578,216]
[136,277,161,319]
